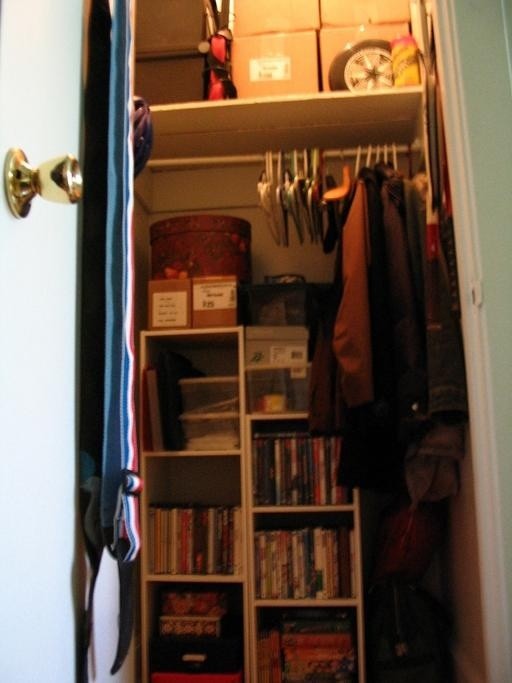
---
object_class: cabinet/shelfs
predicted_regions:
[138,328,367,683]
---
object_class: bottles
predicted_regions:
[389,35,422,87]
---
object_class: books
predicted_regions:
[248,427,360,681]
[143,497,245,584]
[143,367,166,453]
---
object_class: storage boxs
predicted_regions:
[246,370,310,413]
[177,412,239,451]
[279,619,357,683]
[147,281,191,328]
[156,586,240,682]
[178,375,239,413]
[150,215,252,277]
[135,0,419,108]
[246,325,309,362]
[193,277,238,328]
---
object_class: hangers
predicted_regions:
[252,140,415,254]
